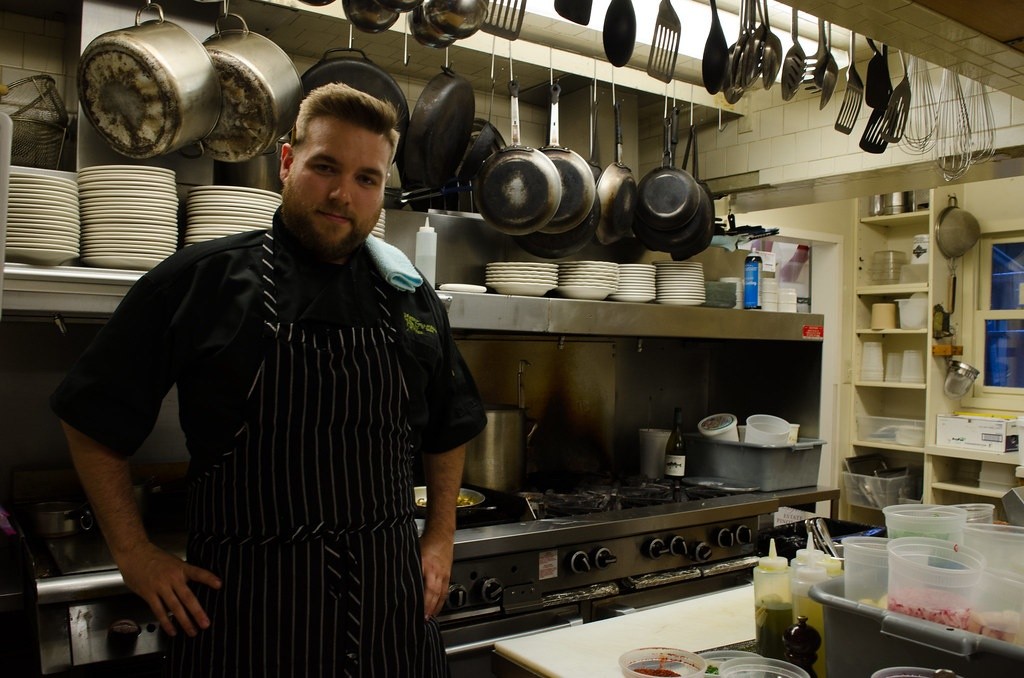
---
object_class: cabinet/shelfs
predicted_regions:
[840,176,1024,529]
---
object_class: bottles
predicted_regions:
[415,217,437,290]
[665,411,686,483]
[743,247,762,308]
[754,532,841,678]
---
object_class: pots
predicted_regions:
[77,3,304,162]
[288,48,410,165]
[405,65,715,260]
[413,401,540,523]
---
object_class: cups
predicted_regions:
[761,278,797,312]
[697,651,767,678]
[860,342,925,383]
[720,277,743,309]
[882,503,1024,648]
[718,657,811,678]
[871,667,965,678]
[618,647,707,678]
[636,413,800,478]
[842,535,929,610]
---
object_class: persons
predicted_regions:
[60,80,489,678]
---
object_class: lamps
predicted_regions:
[490,0,848,83]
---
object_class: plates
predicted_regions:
[5,165,386,271]
[486,262,737,306]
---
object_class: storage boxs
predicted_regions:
[936,413,1023,453]
[681,432,827,491]
[894,299,928,329]
[841,469,916,510]
[857,411,925,447]
[807,576,1024,678]
